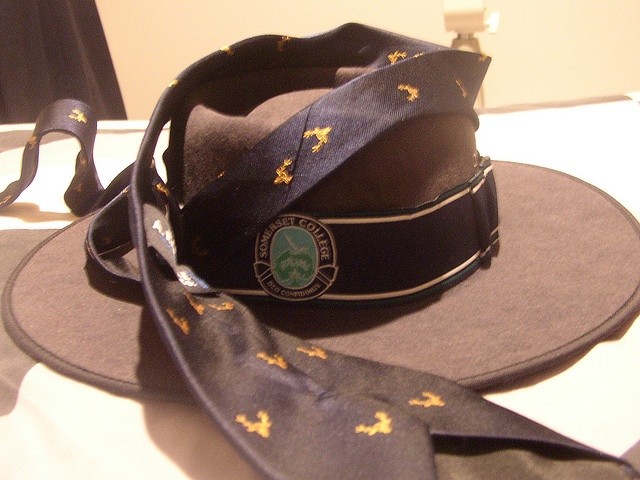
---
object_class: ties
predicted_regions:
[0,21,640,479]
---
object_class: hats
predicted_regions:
[0,63,640,402]
